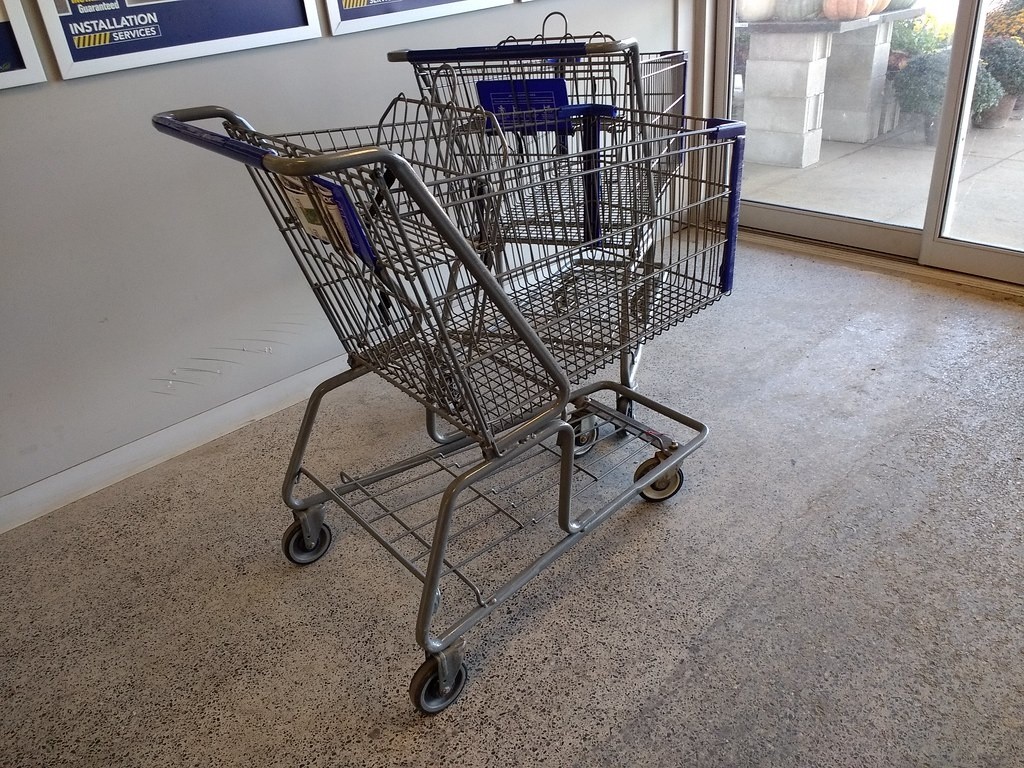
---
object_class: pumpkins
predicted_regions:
[736,0,916,22]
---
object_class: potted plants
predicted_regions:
[985,1,1024,110]
[893,36,1024,146]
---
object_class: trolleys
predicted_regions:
[149,83,747,717]
[384,10,696,440]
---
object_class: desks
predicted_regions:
[743,8,928,167]
